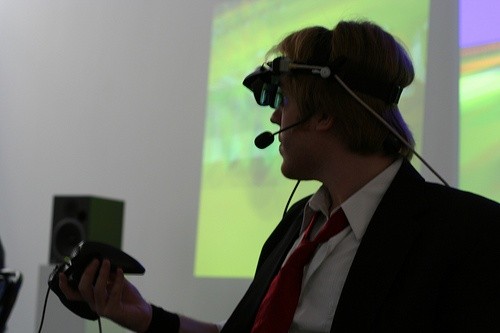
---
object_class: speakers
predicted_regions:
[49,196,122,264]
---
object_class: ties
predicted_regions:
[250,208,349,333]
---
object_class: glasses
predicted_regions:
[254,74,282,109]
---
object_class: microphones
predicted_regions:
[254,111,312,149]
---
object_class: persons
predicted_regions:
[59,19,499,332]
